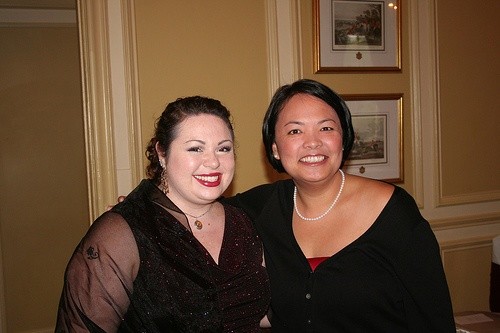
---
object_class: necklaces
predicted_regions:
[184,204,212,231]
[294,168,345,222]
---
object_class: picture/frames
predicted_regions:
[312,0,402,73]
[339,93,404,185]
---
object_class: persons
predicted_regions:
[107,79,457,333]
[56,96,271,333]
